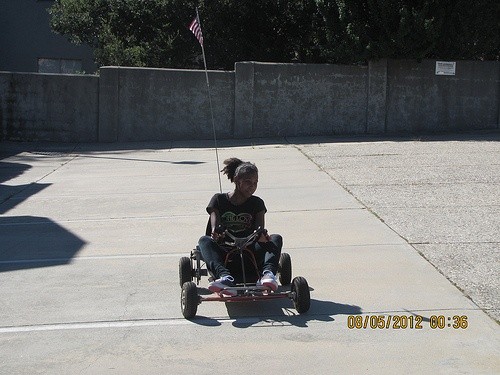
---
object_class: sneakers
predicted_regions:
[208,275,237,296]
[257,272,278,292]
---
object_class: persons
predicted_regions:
[198,156,283,296]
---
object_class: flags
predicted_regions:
[189,15,204,45]
